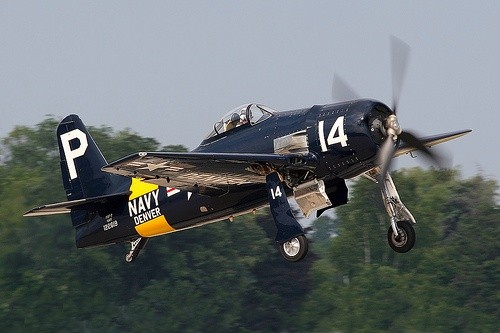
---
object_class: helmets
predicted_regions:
[231,113,240,122]
[239,107,247,117]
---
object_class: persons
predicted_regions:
[226,113,240,131]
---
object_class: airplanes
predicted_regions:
[21,98,473,261]
